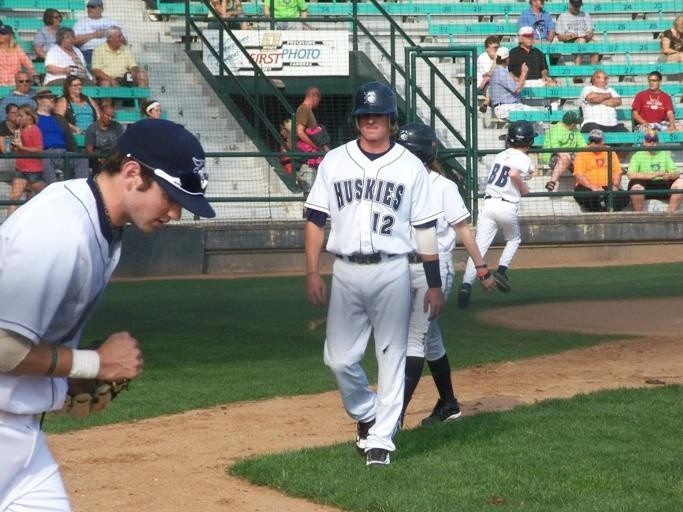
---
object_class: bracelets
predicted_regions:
[474,265,486,268]
[70,349,98,380]
[422,260,442,288]
[304,271,318,277]
[47,340,56,378]
[476,271,490,281]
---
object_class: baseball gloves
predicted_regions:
[61,341,130,414]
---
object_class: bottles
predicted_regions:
[6,137,12,152]
[124,67,135,85]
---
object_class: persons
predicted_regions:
[280,86,330,190]
[0,118,217,512]
[304,81,443,468]
[207,0,309,30]
[1,0,161,214]
[477,0,683,217]
[391,122,500,441]
[457,121,539,306]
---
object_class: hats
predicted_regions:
[87,0,103,8]
[496,47,509,60]
[30,89,59,99]
[562,113,584,124]
[645,131,658,141]
[518,26,536,35]
[117,120,216,218]
[568,0,582,6]
[589,130,604,142]
[0,25,12,34]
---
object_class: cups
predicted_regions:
[70,65,79,76]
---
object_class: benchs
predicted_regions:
[0,2,167,150]
[150,0,682,27]
[427,28,683,205]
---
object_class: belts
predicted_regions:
[336,254,394,264]
[408,253,422,264]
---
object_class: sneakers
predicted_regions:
[479,105,487,112]
[459,282,471,308]
[493,270,510,292]
[423,398,461,425]
[365,448,390,466]
[355,417,376,451]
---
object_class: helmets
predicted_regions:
[352,82,399,131]
[396,123,437,166]
[508,119,540,148]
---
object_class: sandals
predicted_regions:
[546,179,556,191]
